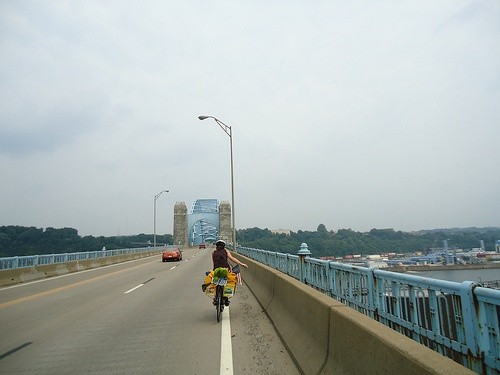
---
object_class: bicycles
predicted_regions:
[213,286,230,322]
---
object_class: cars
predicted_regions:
[162,246,183,262]
[199,243,206,249]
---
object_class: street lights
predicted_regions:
[154,189,169,248]
[198,116,237,252]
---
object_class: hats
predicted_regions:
[216,240,226,245]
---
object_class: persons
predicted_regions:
[211,240,248,307]
